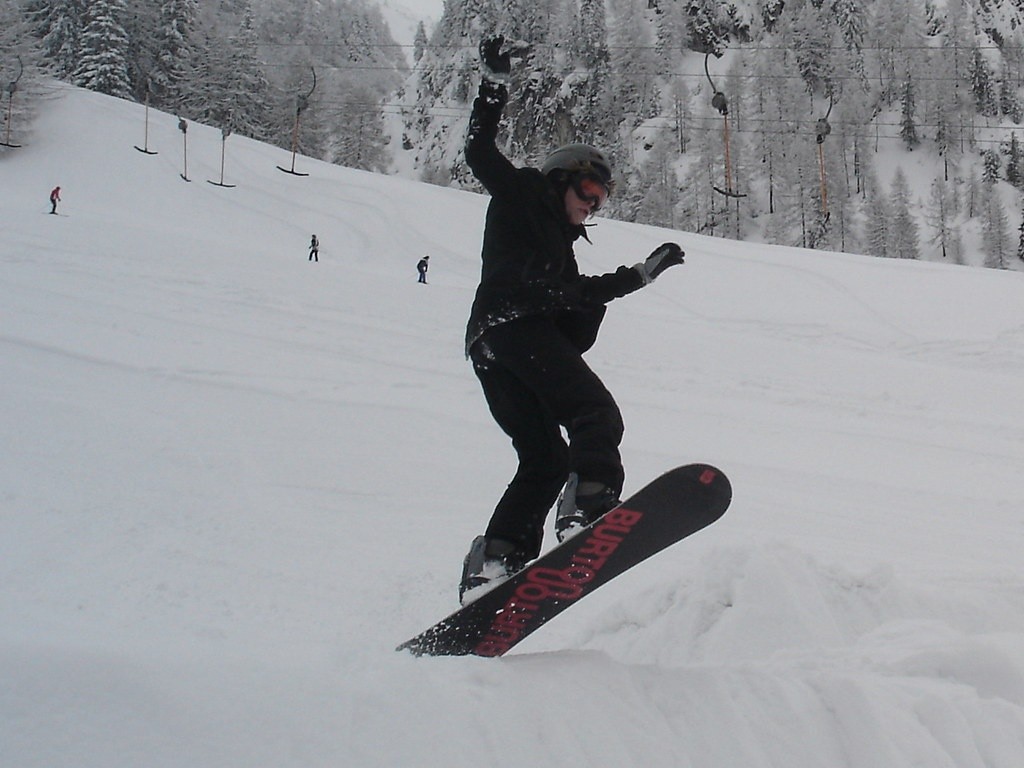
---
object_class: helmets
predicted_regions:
[541,143,611,183]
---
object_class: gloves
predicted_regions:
[479,34,511,84]
[635,243,685,285]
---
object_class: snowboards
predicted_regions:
[394,462,734,658]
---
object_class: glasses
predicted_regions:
[573,173,610,211]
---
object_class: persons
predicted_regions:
[50,187,60,213]
[309,234,319,261]
[417,256,430,283]
[458,34,686,604]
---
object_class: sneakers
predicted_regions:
[459,535,537,606]
[555,472,623,543]
